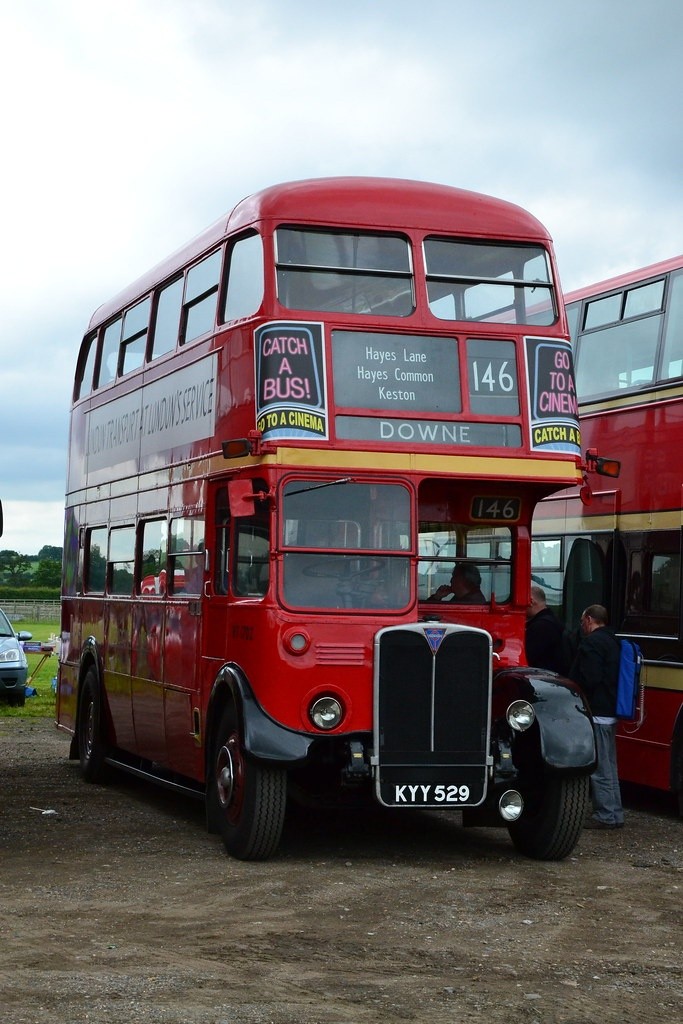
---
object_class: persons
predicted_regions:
[566,605,622,830]
[422,562,488,605]
[524,586,568,676]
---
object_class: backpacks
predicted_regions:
[591,629,642,720]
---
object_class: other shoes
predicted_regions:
[583,817,624,829]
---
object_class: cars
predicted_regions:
[0,609,32,708]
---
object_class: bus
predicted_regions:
[283,253,683,819]
[53,176,597,861]
